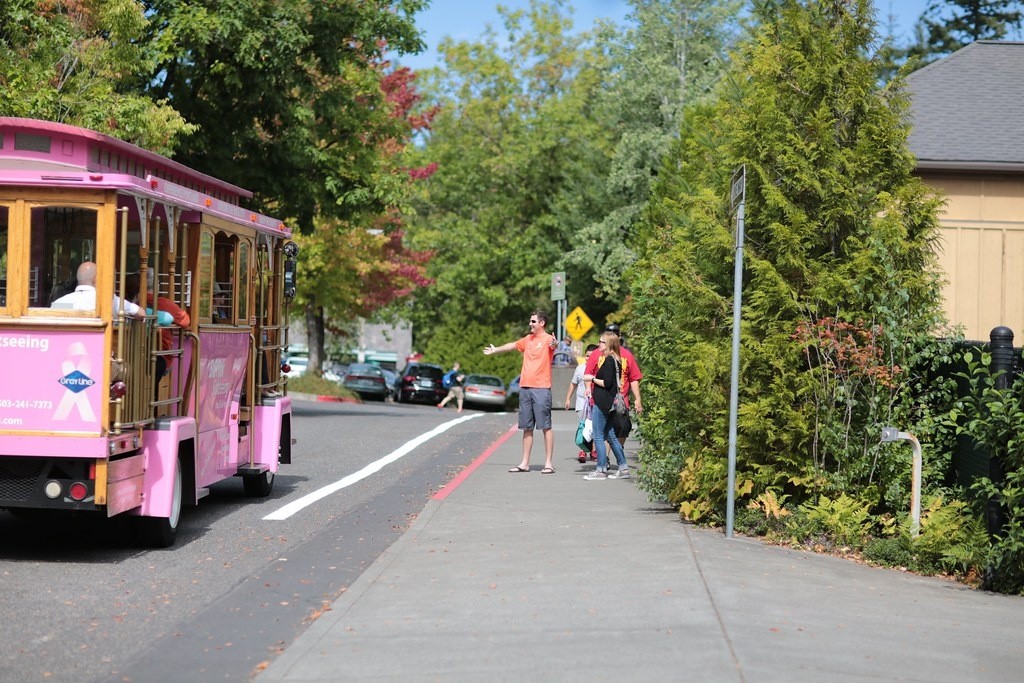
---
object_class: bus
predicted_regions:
[1,116,299,548]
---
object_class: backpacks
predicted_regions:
[443,371,456,388]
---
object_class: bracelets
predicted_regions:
[592,378,595,383]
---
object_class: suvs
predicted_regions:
[394,363,449,407]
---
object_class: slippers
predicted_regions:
[509,466,529,472]
[541,467,555,474]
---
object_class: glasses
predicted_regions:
[532,320,539,323]
[599,341,605,343]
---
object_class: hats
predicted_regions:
[582,418,593,442]
[606,324,620,336]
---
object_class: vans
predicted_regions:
[282,357,341,385]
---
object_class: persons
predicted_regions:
[147,268,190,418]
[114,273,173,335]
[552,335,574,365]
[437,362,465,413]
[212,282,282,406]
[48,269,75,302]
[50,260,146,383]
[483,311,559,473]
[565,324,642,480]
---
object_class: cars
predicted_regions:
[462,374,508,412]
[381,369,397,394]
[510,376,523,398]
[344,364,388,402]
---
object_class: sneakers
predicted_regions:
[583,471,606,480]
[608,469,630,479]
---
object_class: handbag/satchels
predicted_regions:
[614,392,626,416]
[575,397,594,452]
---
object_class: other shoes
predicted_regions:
[606,457,610,469]
[437,404,443,411]
[457,411,461,414]
[578,451,586,463]
[590,450,598,462]
